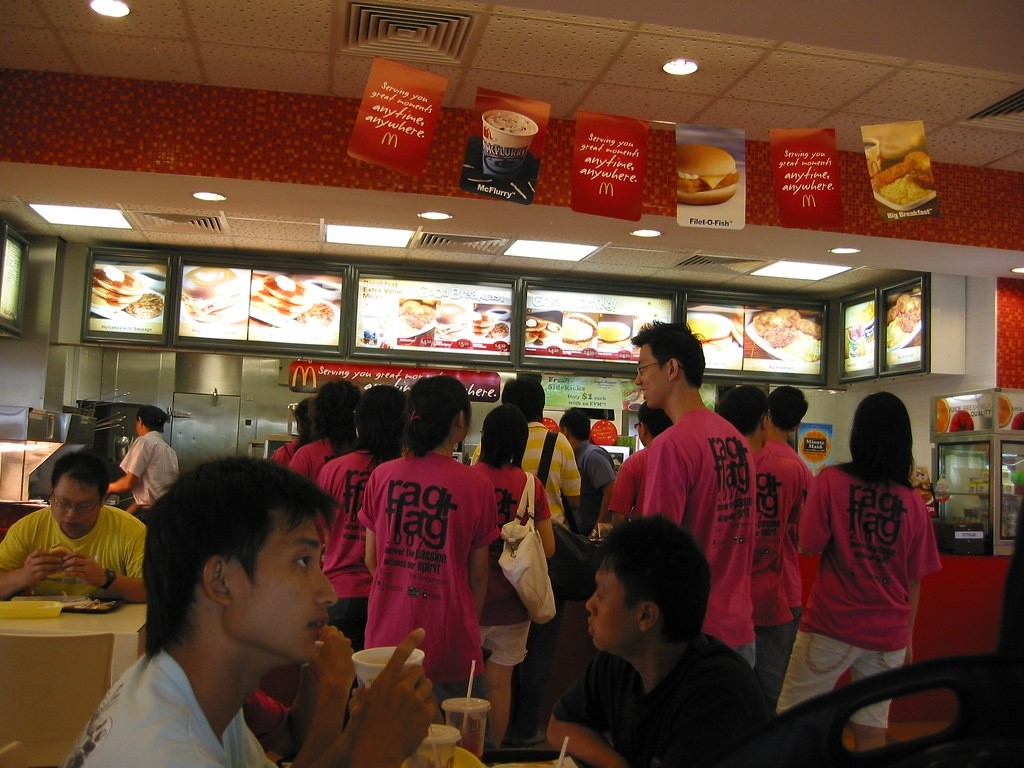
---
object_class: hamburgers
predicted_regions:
[182,267,237,323]
[46,543,74,578]
[435,304,466,335]
[677,145,738,205]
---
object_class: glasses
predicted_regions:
[636,360,680,376]
[634,422,642,430]
[48,487,102,515]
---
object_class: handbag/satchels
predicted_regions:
[543,520,593,597]
[498,474,556,623]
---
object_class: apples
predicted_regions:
[950,410,974,432]
[1011,412,1024,430]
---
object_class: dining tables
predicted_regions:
[0,602,147,660]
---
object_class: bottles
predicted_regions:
[968,465,1024,537]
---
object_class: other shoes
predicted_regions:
[503,728,546,747]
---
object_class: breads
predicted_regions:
[402,299,435,329]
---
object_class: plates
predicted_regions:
[745,322,807,360]
[251,298,341,344]
[1,601,63,619]
[687,312,734,339]
[397,310,435,338]
[874,188,936,211]
[90,290,165,325]
[887,320,922,350]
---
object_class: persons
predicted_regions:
[63,453,437,768]
[355,374,500,751]
[270,397,312,466]
[607,401,674,529]
[468,404,557,746]
[0,449,149,607]
[546,508,772,768]
[240,618,357,768]
[102,405,180,526]
[610,322,757,669]
[715,385,797,708]
[313,384,406,728]
[501,376,582,744]
[762,387,815,627]
[559,406,618,544]
[287,380,361,570]
[798,390,941,751]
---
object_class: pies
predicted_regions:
[753,309,821,348]
[887,294,921,333]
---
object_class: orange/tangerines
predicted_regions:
[999,394,1013,428]
[936,397,949,432]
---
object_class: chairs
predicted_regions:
[0,632,115,768]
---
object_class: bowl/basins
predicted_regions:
[401,745,485,768]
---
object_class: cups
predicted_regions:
[414,724,457,768]
[352,646,425,727]
[847,333,858,358]
[492,762,564,767]
[628,403,643,411]
[862,325,874,342]
[863,137,882,177]
[305,279,342,302]
[133,270,166,291]
[487,309,510,321]
[482,110,539,181]
[441,697,492,762]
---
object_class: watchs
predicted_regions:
[590,529,598,539]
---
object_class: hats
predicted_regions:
[137,404,168,432]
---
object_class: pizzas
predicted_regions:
[486,323,510,339]
[298,304,334,325]
[125,293,163,318]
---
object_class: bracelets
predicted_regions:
[99,568,116,591]
